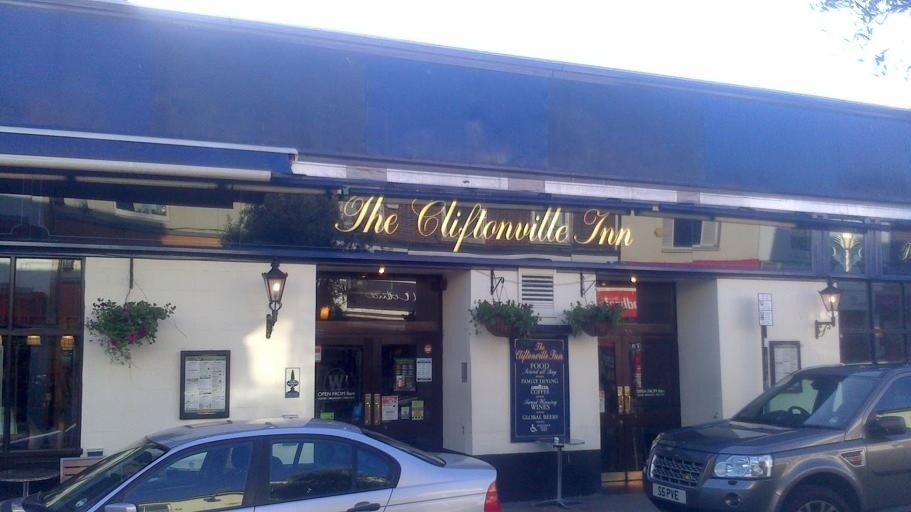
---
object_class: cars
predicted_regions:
[0,416,498,511]
[639,359,910,512]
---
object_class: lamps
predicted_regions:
[814,279,843,341]
[260,255,288,340]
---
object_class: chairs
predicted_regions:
[224,446,249,495]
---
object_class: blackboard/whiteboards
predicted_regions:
[509,336,570,444]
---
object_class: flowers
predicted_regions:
[466,296,542,342]
[83,295,175,367]
[561,298,626,339]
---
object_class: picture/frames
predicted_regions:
[769,339,803,394]
[178,348,232,420]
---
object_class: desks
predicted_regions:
[531,437,587,511]
